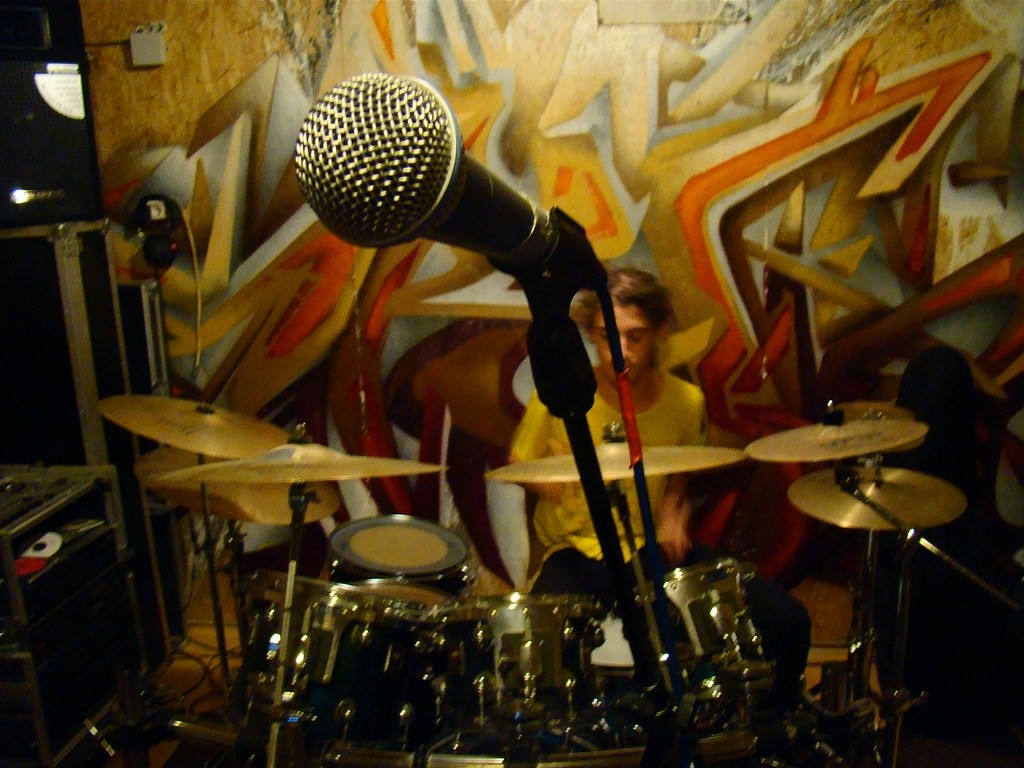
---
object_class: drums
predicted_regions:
[229,511,779,751]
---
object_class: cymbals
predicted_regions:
[484,441,746,482]
[785,462,969,532]
[93,394,305,464]
[746,419,931,464]
[820,400,914,427]
[150,440,450,485]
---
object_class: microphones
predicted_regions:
[293,71,608,297]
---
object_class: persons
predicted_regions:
[508,263,814,696]
